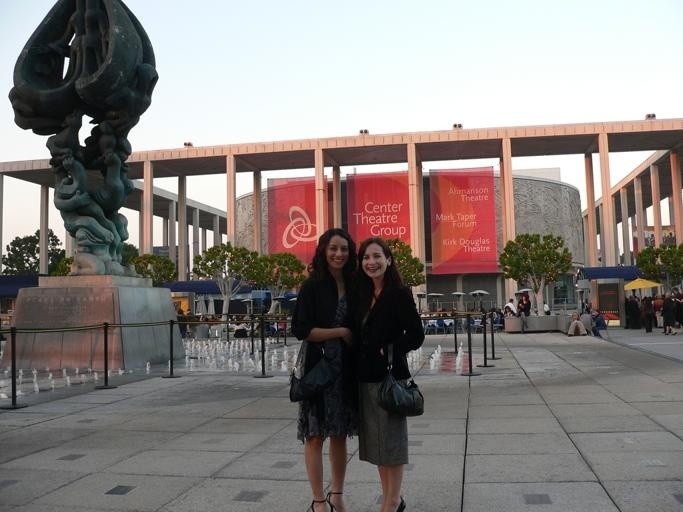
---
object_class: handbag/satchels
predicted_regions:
[375,346,424,416]
[289,348,341,402]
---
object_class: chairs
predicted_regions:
[577,313,591,336]
[422,313,503,335]
[592,317,610,337]
[177,308,294,340]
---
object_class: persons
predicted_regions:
[572,308,606,336]
[177,308,250,338]
[625,287,683,335]
[490,293,551,317]
[354,237,425,512]
[290,229,360,512]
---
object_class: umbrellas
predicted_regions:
[624,277,664,291]
